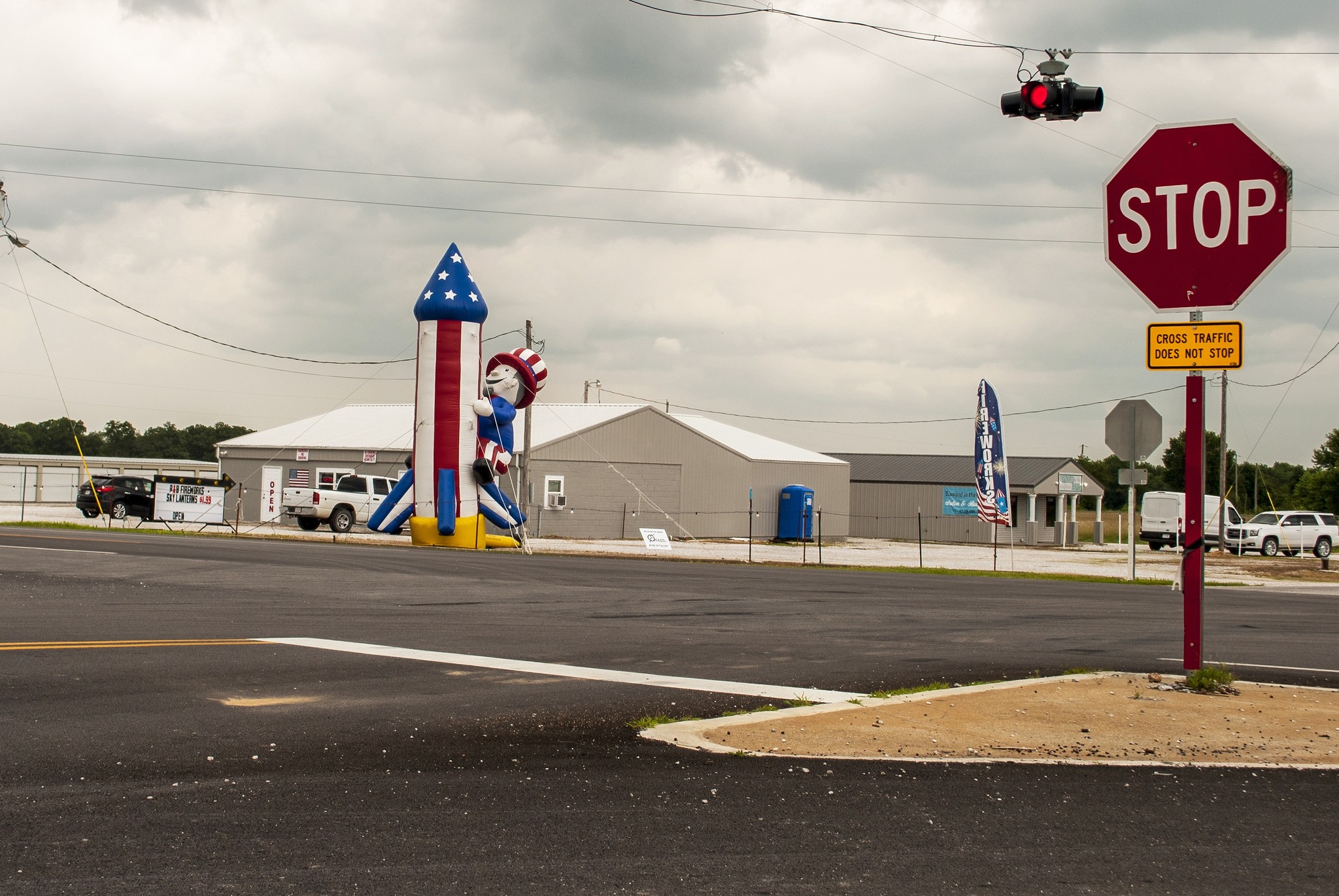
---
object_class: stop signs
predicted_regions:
[1103,117,1294,314]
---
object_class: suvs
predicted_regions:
[1225,510,1339,558]
[76,473,154,522]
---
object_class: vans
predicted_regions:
[1139,491,1247,555]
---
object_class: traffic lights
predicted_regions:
[1020,79,1062,115]
[1001,85,1024,118]
[1061,83,1104,117]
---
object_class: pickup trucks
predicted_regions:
[281,473,390,529]
